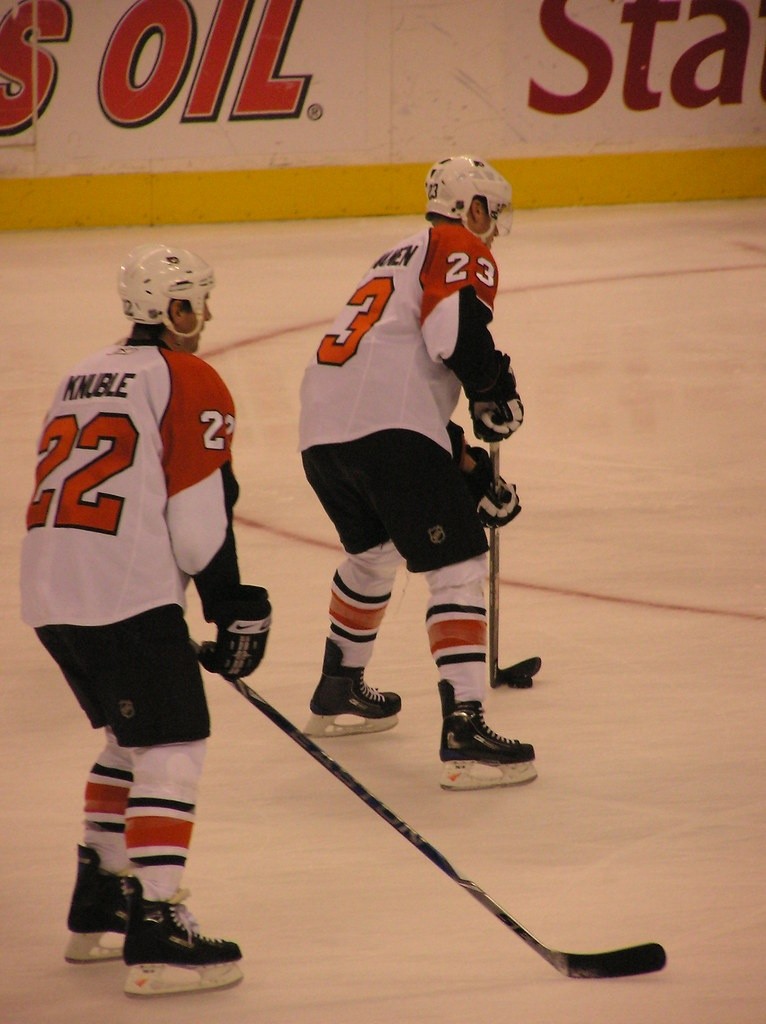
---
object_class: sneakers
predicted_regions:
[121,875,245,995]
[302,636,402,738]
[63,842,133,964]
[437,678,537,792]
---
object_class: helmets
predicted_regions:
[116,243,216,337]
[423,154,513,243]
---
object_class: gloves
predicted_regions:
[198,583,273,682]
[467,447,522,528]
[463,349,525,443]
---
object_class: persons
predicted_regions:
[298,156,535,766]
[20,246,243,966]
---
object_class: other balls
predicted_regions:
[508,676,533,689]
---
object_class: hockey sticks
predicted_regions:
[485,410,544,690]
[190,632,670,982]
